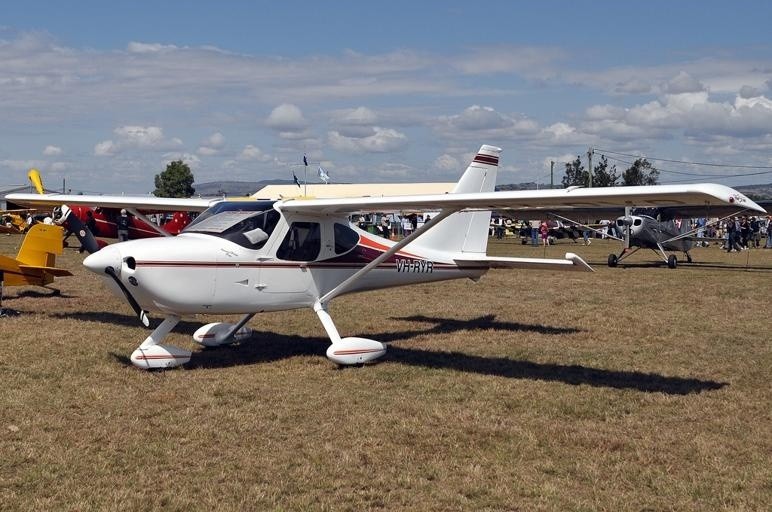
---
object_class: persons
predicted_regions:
[43,214,52,224]
[583,232,591,246]
[381,213,389,238]
[5,215,12,236]
[599,220,617,239]
[118,208,128,242]
[27,213,32,224]
[520,222,528,245]
[401,215,417,238]
[497,216,504,239]
[695,216,772,252]
[541,222,549,247]
[425,215,431,224]
[359,215,366,229]
[79,211,95,254]
